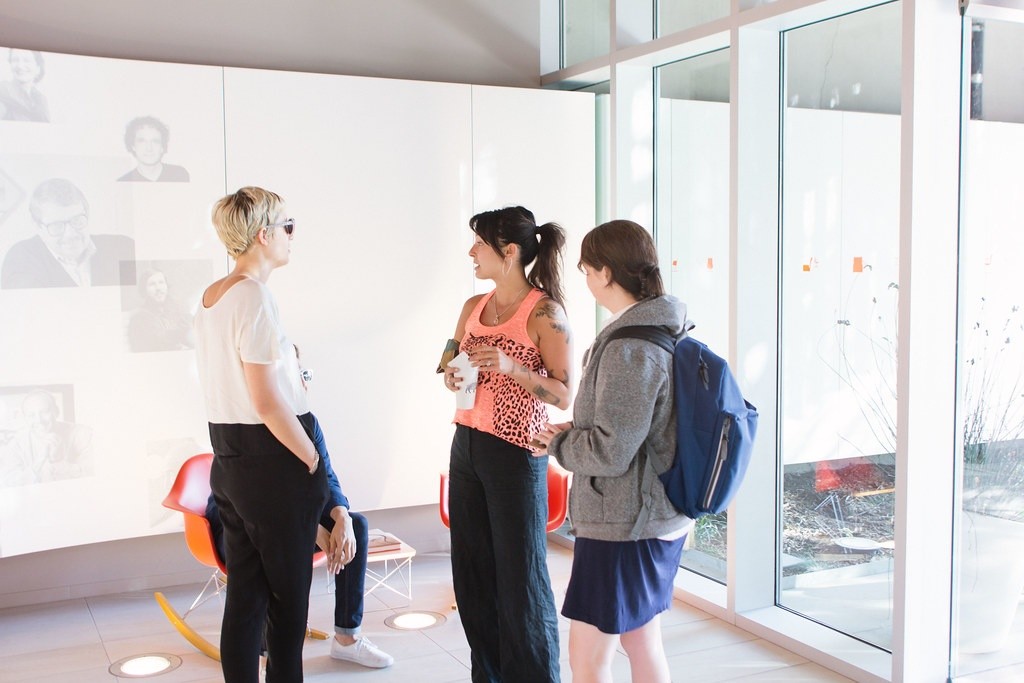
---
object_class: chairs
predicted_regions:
[814,458,876,555]
[439,462,569,612]
[153,453,329,662]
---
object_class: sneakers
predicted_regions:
[330,636,393,668]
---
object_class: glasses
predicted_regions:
[265,218,295,235]
[303,370,313,383]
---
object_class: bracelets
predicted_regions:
[309,448,319,475]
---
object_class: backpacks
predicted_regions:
[594,325,759,520]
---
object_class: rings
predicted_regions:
[446,376,453,385]
[486,360,490,366]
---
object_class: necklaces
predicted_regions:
[493,287,522,325]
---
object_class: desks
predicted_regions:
[327,528,416,614]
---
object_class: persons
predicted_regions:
[531,220,699,683]
[0,53,199,483]
[206,347,393,683]
[195,187,326,683]
[441,206,577,683]
